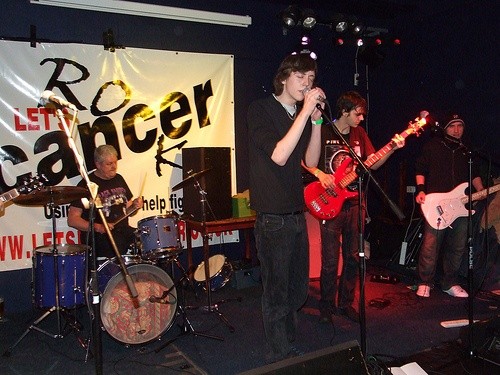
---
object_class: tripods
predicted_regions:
[429,130,500,375]
[3,188,94,359]
[155,177,235,354]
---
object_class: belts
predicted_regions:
[263,209,304,215]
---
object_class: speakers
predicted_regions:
[239,339,370,375]
[182,147,233,223]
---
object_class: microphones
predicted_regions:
[302,88,325,102]
[149,295,169,304]
[421,111,440,127]
[42,91,77,111]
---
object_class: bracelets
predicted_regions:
[415,184,425,193]
[311,117,324,125]
[314,169,321,177]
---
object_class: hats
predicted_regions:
[443,113,465,130]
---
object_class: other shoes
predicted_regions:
[320,314,335,339]
[443,286,469,297]
[286,346,304,362]
[416,284,430,297]
[339,306,359,322]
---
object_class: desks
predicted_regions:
[182,215,257,310]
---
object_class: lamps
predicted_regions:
[301,7,317,29]
[328,12,348,33]
[281,5,301,30]
[347,15,362,33]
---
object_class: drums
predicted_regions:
[86,254,181,347]
[30,243,94,311]
[194,254,234,292]
[138,214,183,262]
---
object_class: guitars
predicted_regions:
[419,182,500,231]
[303,117,427,222]
[0,172,49,206]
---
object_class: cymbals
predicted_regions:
[12,185,89,207]
[171,167,215,192]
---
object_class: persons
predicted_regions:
[246,53,326,363]
[66,144,144,259]
[301,91,405,323]
[415,115,487,298]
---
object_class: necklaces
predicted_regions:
[272,93,297,120]
